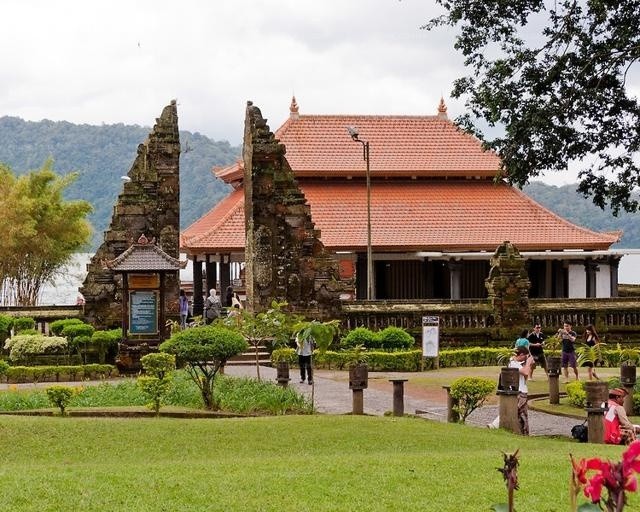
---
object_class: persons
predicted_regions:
[295,330,314,385]
[179,286,242,333]
[486,321,640,445]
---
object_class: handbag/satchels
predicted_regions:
[231,292,244,309]
[571,425,588,441]
[207,309,217,319]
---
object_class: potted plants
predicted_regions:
[542,336,563,374]
[345,343,369,386]
[496,345,520,391]
[575,342,609,408]
[619,345,640,383]
[270,344,299,378]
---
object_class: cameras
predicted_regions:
[525,356,536,370]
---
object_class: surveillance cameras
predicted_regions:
[348,127,359,138]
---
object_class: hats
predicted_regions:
[609,388,625,397]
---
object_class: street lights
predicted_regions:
[347,126,379,300]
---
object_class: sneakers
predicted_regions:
[300,379,313,385]
